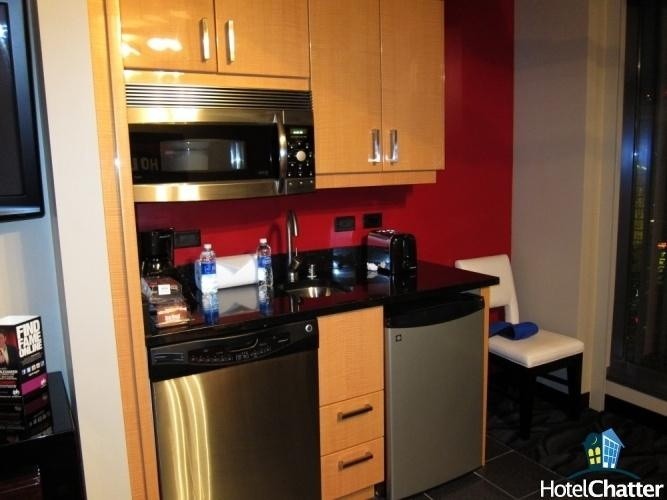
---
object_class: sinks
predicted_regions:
[277,278,350,298]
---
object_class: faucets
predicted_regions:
[286,208,301,283]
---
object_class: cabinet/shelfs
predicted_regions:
[118,1,445,191]
[316,305,384,500]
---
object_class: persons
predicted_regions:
[0,328,19,370]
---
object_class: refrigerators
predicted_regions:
[147,317,322,500]
[379,289,484,500]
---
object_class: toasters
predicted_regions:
[362,227,421,277]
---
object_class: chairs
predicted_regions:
[454,254,584,441]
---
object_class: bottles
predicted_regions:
[201,291,219,325]
[258,286,275,318]
[199,243,219,294]
[256,238,275,288]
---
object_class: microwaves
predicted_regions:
[123,81,317,204]
